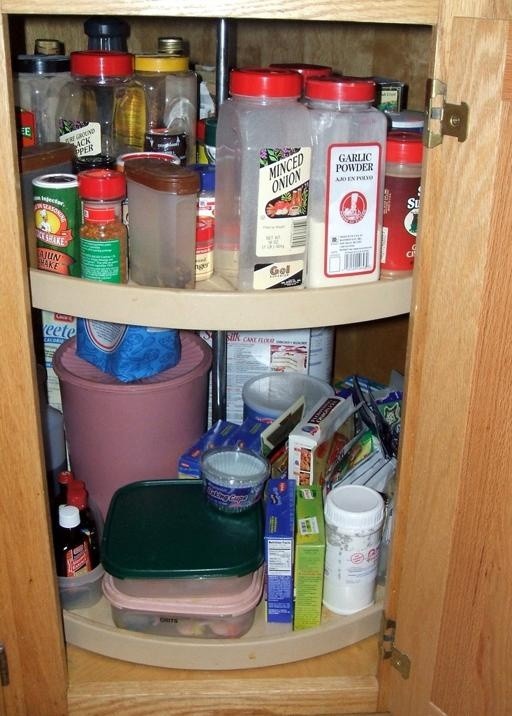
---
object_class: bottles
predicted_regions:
[321,483,386,616]
[16,18,424,291]
[52,469,99,577]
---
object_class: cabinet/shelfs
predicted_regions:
[0,1,439,716]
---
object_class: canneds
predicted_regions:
[380,110,427,279]
[32,173,81,279]
[73,154,117,175]
[144,128,187,168]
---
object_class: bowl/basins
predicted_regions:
[200,450,272,516]
